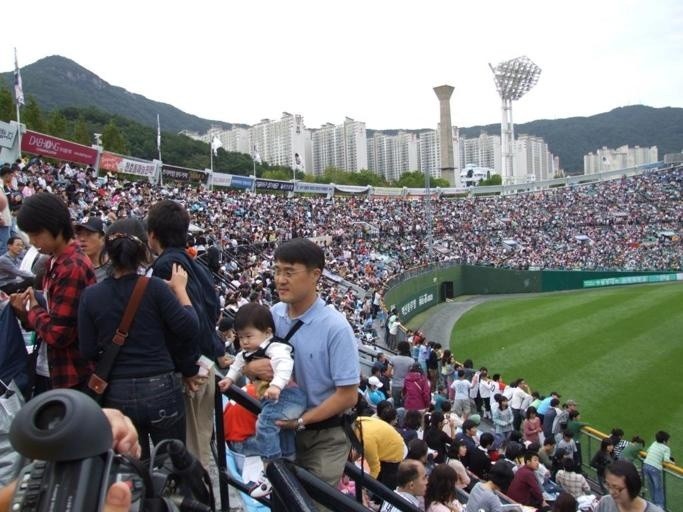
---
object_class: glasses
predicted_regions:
[270,267,313,278]
[601,481,626,494]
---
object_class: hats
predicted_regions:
[550,392,578,405]
[72,216,109,235]
[368,375,384,388]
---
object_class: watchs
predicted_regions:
[292,416,307,434]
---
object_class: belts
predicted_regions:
[303,413,359,430]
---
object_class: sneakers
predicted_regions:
[247,476,272,498]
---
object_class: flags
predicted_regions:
[291,153,296,170]
[253,145,262,166]
[210,132,223,157]
[14,61,25,111]
[157,114,161,151]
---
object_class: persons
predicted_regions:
[7,161,242,470]
[0,407,142,511]
[243,237,361,512]
[246,164,682,322]
[336,324,674,512]
[218,302,307,499]
